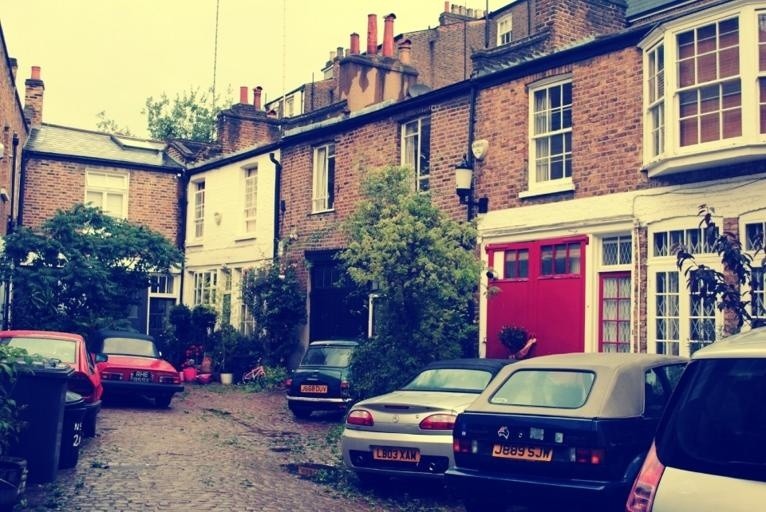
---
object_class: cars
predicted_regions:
[285,341,360,418]
[340,357,558,506]
[444,352,691,512]
[0,330,185,437]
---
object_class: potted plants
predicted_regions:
[178,350,232,385]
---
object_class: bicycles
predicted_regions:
[242,357,267,388]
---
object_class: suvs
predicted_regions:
[625,326,766,512]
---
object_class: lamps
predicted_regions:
[451,152,488,214]
